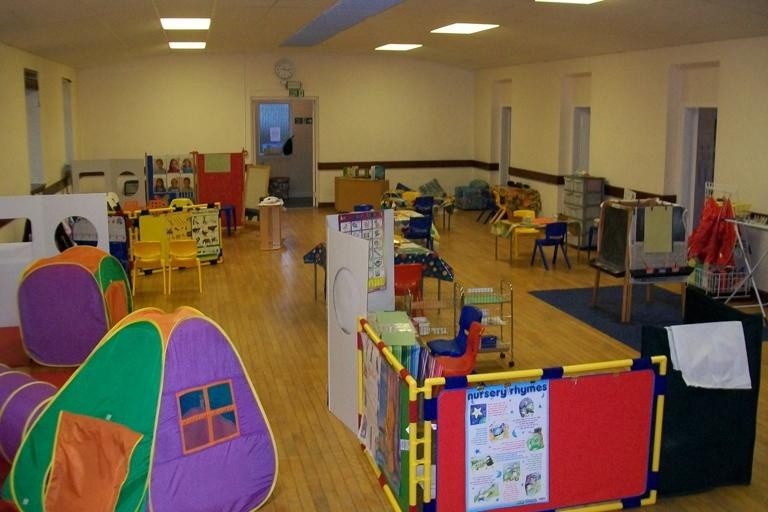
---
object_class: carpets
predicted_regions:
[527,284,683,360]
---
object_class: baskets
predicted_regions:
[693,267,751,293]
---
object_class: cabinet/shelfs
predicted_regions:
[454,281,515,369]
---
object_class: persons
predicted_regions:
[153,178,166,192]
[167,158,180,174]
[154,159,166,174]
[167,178,180,193]
[181,177,194,192]
[182,159,192,174]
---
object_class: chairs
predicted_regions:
[490,190,508,223]
[414,196,433,215]
[531,222,571,272]
[393,265,427,316]
[404,216,434,250]
[512,210,541,258]
[165,238,203,296]
[131,241,168,298]
[426,306,490,395]
[477,190,496,223]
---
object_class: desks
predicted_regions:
[723,218,768,322]
[494,217,581,267]
[256,202,282,249]
[334,176,389,214]
[393,209,440,251]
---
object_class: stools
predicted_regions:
[220,204,237,236]
